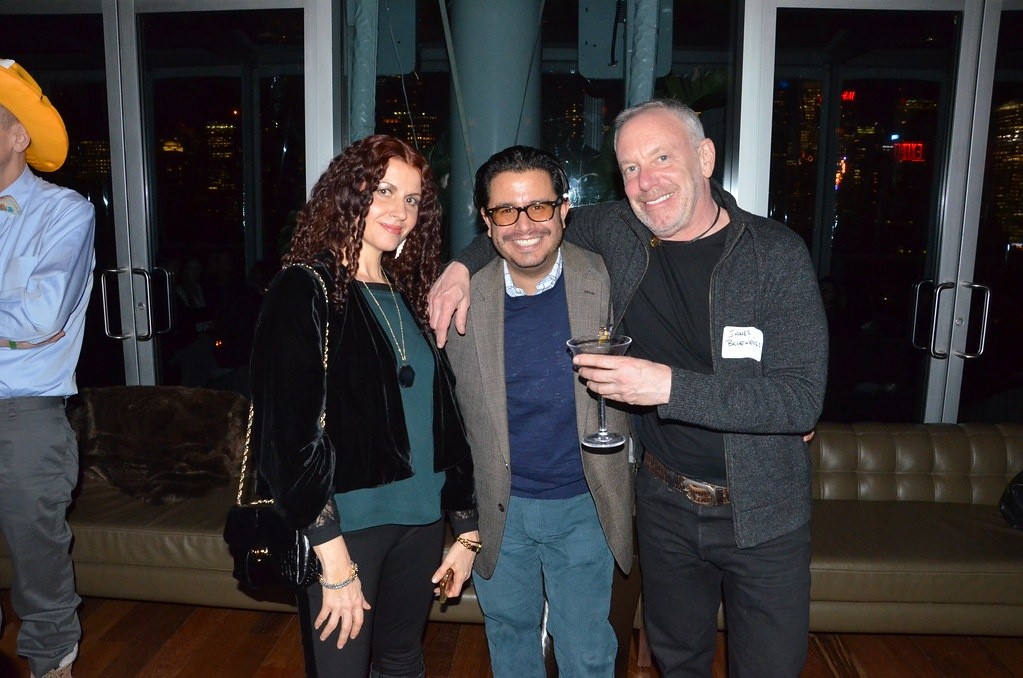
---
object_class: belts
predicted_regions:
[641,449,734,507]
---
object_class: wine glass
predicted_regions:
[566,334,631,450]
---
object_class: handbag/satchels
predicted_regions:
[220,499,329,607]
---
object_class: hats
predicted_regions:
[0,53,70,172]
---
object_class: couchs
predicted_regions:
[1,385,485,622]
[632,422,1023,667]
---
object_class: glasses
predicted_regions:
[483,196,564,227]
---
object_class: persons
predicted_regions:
[427,100,829,678]
[0,56,96,678]
[443,144,815,678]
[247,134,479,678]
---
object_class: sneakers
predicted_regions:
[31,651,74,676]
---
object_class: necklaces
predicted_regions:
[650,201,720,248]
[357,266,416,388]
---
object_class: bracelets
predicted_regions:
[9,340,17,349]
[318,560,358,590]
[457,536,481,553]
